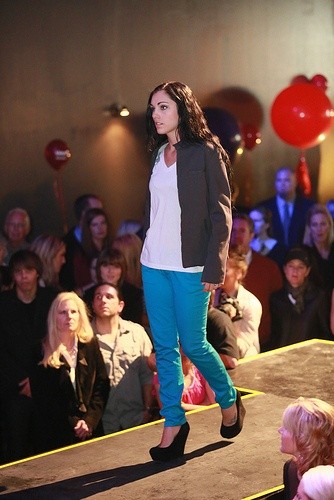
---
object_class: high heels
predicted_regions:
[220,389,246,439]
[150,420,190,463]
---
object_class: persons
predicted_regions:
[0,250,57,466]
[278,397,334,500]
[140,81,246,462]
[26,292,110,455]
[270,248,333,350]
[301,206,334,289]
[250,207,290,271]
[212,244,263,357]
[255,167,314,256]
[90,283,154,436]
[137,290,242,418]
[82,249,143,324]
[0,194,154,309]
[229,214,283,353]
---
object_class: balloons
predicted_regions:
[292,74,329,92]
[198,106,244,167]
[45,139,72,171]
[270,84,334,151]
[242,126,260,152]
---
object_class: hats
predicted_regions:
[284,245,311,268]
